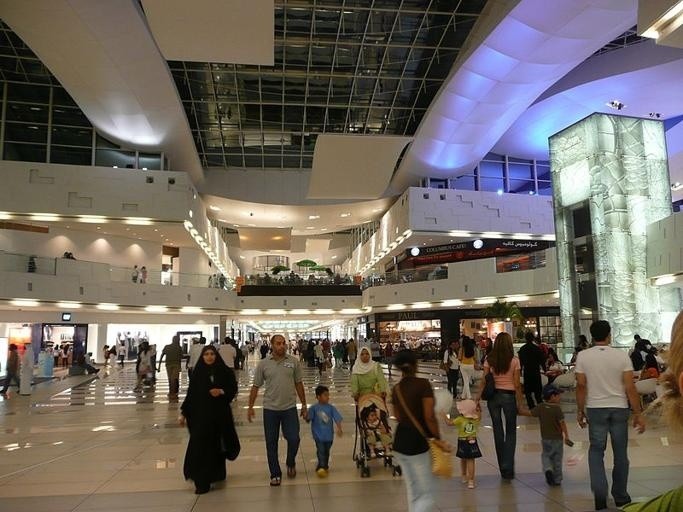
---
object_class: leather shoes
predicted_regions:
[195,486,211,495]
[614,499,632,507]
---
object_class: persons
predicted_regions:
[518,332,546,409]
[484,333,524,479]
[140,267,147,283]
[158,336,183,399]
[517,385,569,486]
[534,338,548,356]
[178,346,241,494]
[103,345,110,367]
[133,341,155,392]
[578,335,591,350]
[350,347,387,406]
[232,339,273,370]
[455,334,493,360]
[385,341,394,374]
[84,352,96,367]
[53,345,60,367]
[59,345,64,353]
[0,344,20,394]
[394,340,439,362]
[458,336,479,400]
[208,273,226,289]
[247,335,308,486]
[630,346,646,371]
[109,346,117,368]
[118,343,127,368]
[440,399,482,489]
[185,337,206,381]
[303,386,344,478]
[288,337,356,375]
[645,347,660,376]
[62,252,74,260]
[362,337,385,356]
[443,339,460,399]
[219,337,236,371]
[243,270,418,290]
[78,350,100,374]
[573,320,646,510]
[571,347,582,363]
[634,334,653,354]
[619,309,683,511]
[363,409,393,458]
[544,352,568,385]
[61,346,69,365]
[209,338,221,351]
[28,254,39,274]
[392,349,441,512]
[132,265,139,283]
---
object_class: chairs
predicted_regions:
[635,378,658,411]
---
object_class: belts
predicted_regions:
[496,387,517,395]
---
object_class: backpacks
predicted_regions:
[395,382,454,481]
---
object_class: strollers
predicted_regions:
[353,391,405,479]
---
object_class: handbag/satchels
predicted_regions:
[481,367,496,402]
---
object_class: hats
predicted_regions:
[543,384,565,396]
[455,399,480,420]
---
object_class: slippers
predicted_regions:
[286,465,297,479]
[270,473,282,486]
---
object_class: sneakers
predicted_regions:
[546,470,554,486]
[461,474,466,486]
[468,480,476,489]
[317,468,328,479]
